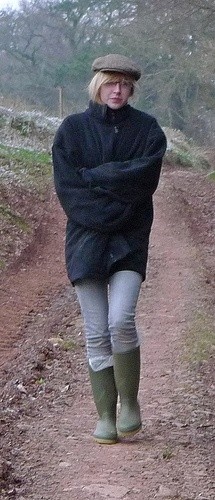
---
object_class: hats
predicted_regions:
[91,54,141,81]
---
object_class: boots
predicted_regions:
[88,345,142,443]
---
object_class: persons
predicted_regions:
[52,54,167,443]
